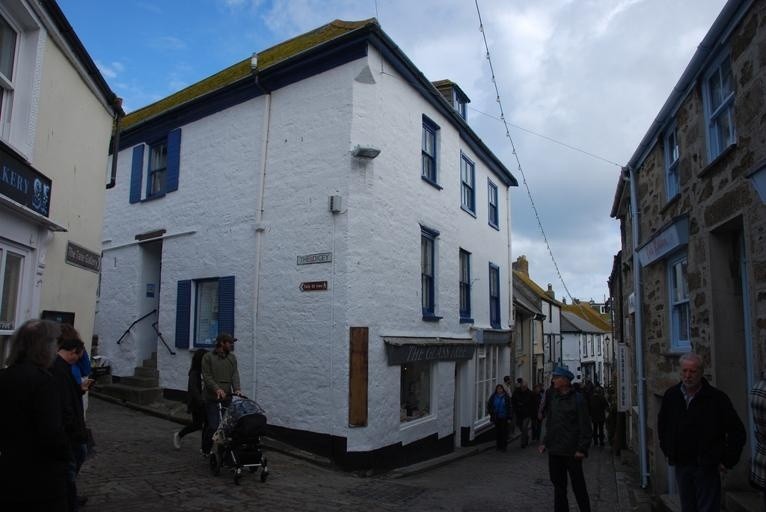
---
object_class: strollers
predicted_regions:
[210,392,269,486]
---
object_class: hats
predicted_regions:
[550,366,575,381]
[215,332,239,345]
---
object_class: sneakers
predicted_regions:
[173,430,185,449]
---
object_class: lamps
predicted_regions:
[353,144,381,159]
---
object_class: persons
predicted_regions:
[538,367,592,511]
[55,323,92,420]
[656,351,747,510]
[0,319,75,511]
[52,335,94,512]
[488,375,609,449]
[173,347,209,451]
[749,372,766,493]
[200,331,241,474]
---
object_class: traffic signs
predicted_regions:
[299,281,327,291]
[65,240,101,274]
